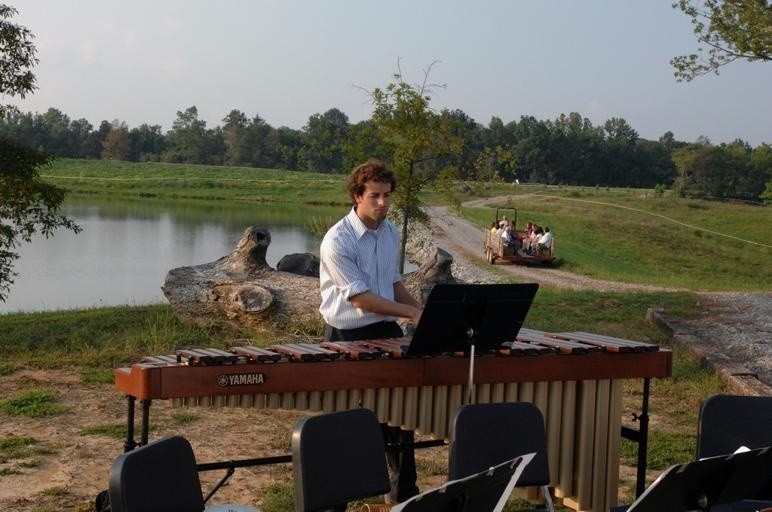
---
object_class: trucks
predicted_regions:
[483,207,556,265]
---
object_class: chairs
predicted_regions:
[95,434,207,511]
[290,407,393,512]
[447,400,559,512]
[694,394,772,460]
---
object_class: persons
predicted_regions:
[491,216,552,256]
[319,158,425,342]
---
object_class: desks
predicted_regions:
[112,328,674,511]
